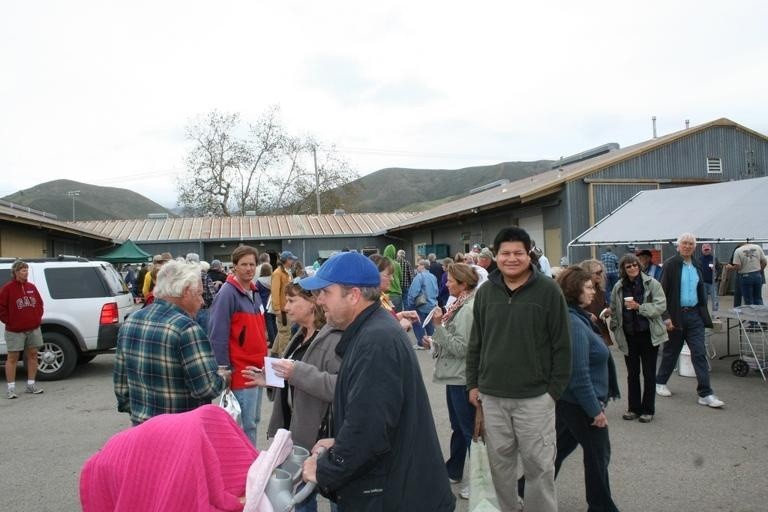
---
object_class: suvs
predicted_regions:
[0,253,136,383]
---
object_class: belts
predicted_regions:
[678,307,696,312]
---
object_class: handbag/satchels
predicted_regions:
[414,293,427,307]
[470,438,502,511]
[220,389,243,428]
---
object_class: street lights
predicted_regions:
[64,189,80,222]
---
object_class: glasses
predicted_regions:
[626,264,638,268]
[292,277,311,295]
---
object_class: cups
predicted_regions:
[623,296,634,311]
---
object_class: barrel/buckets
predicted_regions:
[678,344,697,377]
[704,330,716,369]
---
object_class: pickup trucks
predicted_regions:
[119,262,153,288]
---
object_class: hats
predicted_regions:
[280,251,297,261]
[153,253,199,262]
[299,251,381,290]
[636,250,652,258]
[702,244,711,250]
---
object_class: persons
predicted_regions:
[113,225,622,512]
[0,260,44,399]
[610,232,768,424]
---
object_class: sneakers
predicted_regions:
[639,414,653,422]
[7,389,18,399]
[698,395,724,407]
[623,412,637,420]
[24,384,44,393]
[412,344,425,350]
[656,384,672,397]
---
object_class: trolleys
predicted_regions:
[730,306,767,383]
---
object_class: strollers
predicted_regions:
[78,404,326,512]
